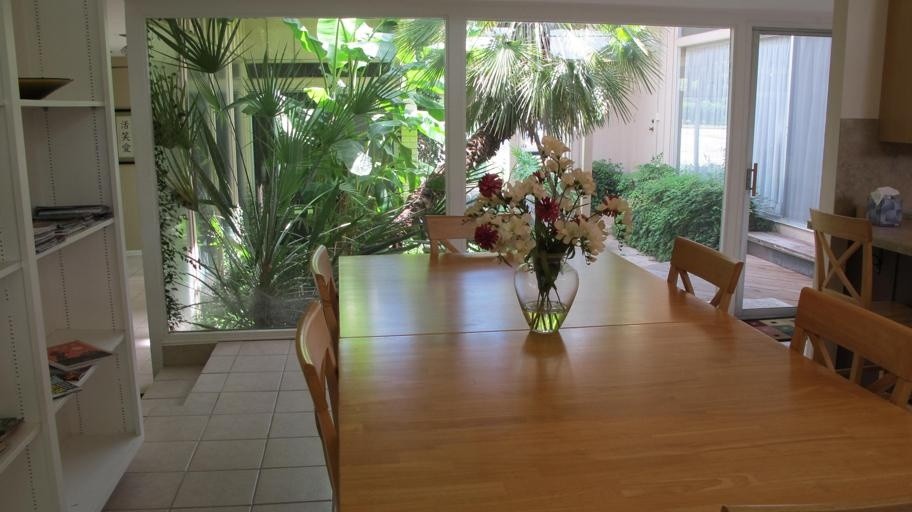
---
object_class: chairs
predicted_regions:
[806,208,911,393]
[294,299,340,509]
[311,243,339,348]
[424,212,532,254]
[665,235,745,311]
[790,286,911,409]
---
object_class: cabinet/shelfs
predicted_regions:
[1,1,143,511]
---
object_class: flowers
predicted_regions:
[461,131,635,332]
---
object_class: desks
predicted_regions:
[338,244,911,508]
[859,215,912,305]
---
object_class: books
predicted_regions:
[32,202,111,255]
[46,339,114,399]
